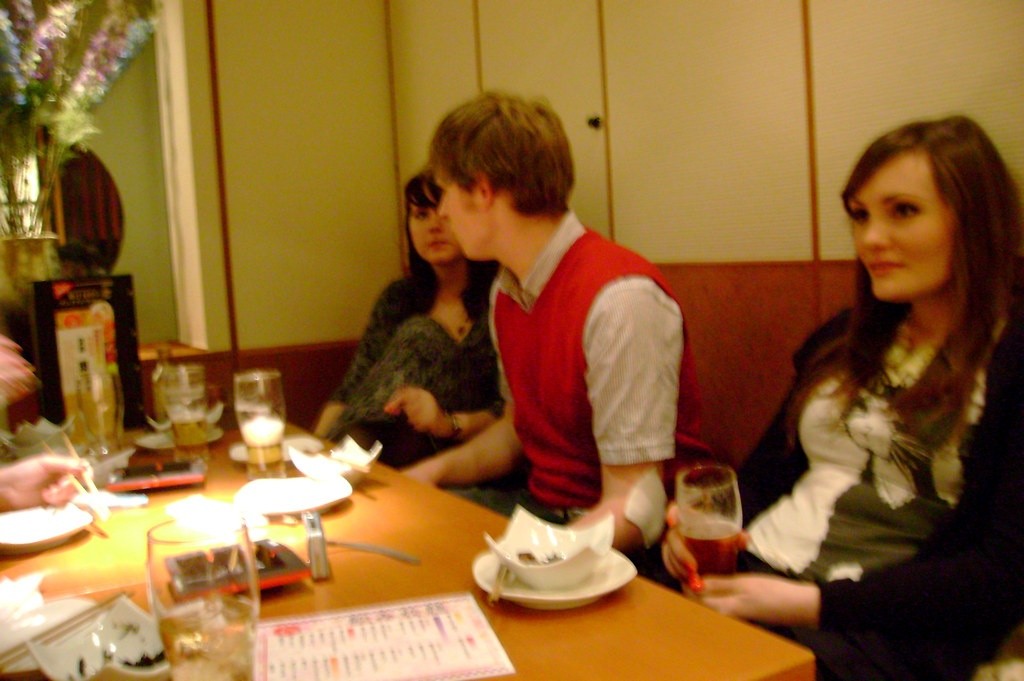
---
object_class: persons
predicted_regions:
[312,161,529,490]
[396,88,723,591]
[0,454,88,512]
[642,116,1024,681]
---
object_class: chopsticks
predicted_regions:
[40,433,112,520]
[0,590,134,670]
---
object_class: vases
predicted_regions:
[0,233,60,311]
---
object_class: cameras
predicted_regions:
[301,510,330,580]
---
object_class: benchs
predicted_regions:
[654,259,863,471]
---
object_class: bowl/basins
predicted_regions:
[2,418,75,457]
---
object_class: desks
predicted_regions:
[0,413,815,681]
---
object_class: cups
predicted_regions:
[163,365,210,462]
[234,371,286,479]
[77,370,121,460]
[146,520,260,680]
[674,465,742,576]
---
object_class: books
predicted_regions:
[252,590,516,681]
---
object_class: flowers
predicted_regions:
[0,0,160,242]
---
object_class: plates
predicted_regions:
[0,507,92,554]
[472,553,637,608]
[484,504,615,587]
[0,598,103,674]
[145,401,224,434]
[132,427,224,451]
[232,476,354,516]
[228,436,323,464]
[27,595,170,681]
[287,434,383,483]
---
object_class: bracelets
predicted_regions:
[447,412,463,440]
